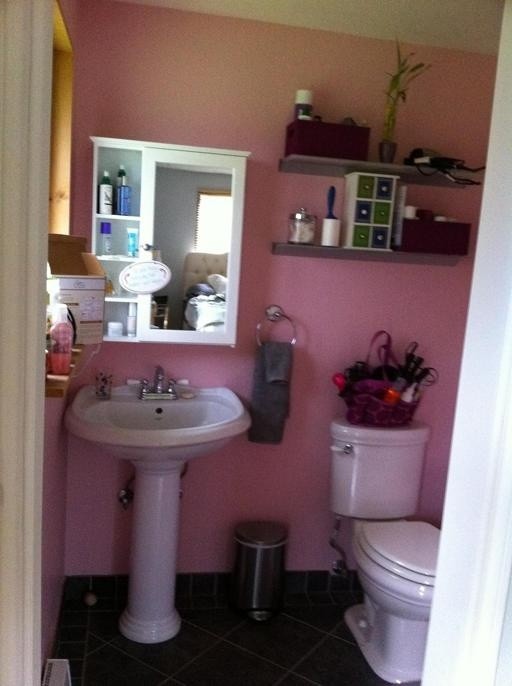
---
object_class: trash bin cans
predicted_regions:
[232,520,288,621]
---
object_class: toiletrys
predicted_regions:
[334,359,425,428]
[98,164,138,257]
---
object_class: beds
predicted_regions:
[180,251,230,332]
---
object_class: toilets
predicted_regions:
[330,416,442,685]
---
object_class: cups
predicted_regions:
[107,321,124,338]
[94,369,113,399]
[127,316,136,336]
[319,218,342,247]
[405,204,432,221]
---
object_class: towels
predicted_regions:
[246,335,293,445]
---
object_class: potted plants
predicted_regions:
[378,25,432,165]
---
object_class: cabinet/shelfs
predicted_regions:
[271,154,469,267]
[90,132,253,341]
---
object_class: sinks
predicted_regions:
[62,383,251,463]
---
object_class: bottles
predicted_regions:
[381,376,417,408]
[105,271,113,297]
[287,207,317,244]
[97,164,131,215]
[294,89,314,120]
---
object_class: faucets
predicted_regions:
[154,364,165,393]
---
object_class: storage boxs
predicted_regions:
[404,216,471,256]
[43,232,107,350]
[286,120,370,161]
[341,172,400,252]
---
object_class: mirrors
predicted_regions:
[135,147,247,348]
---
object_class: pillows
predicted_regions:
[186,271,229,300]
